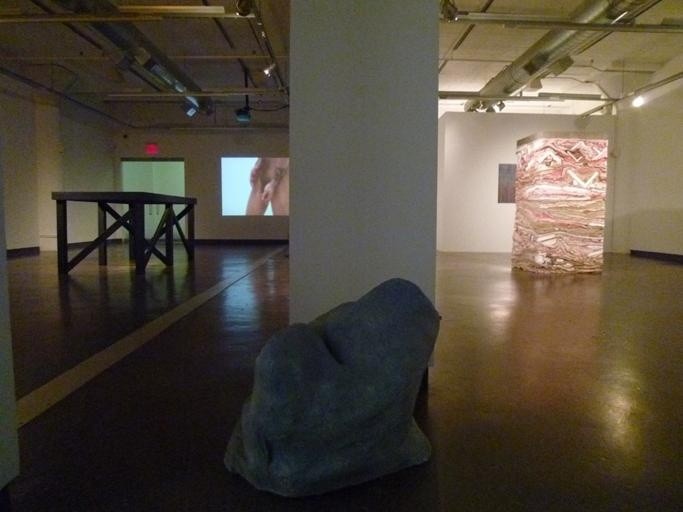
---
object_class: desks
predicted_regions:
[50,190,197,276]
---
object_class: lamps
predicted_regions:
[233,0,284,92]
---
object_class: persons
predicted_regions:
[244,156,289,216]
